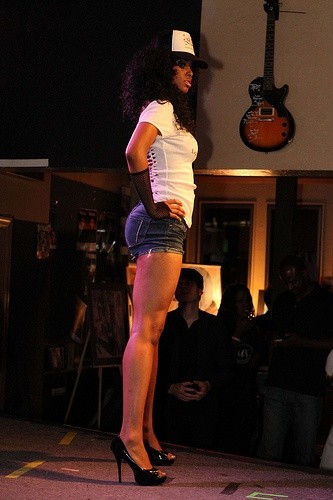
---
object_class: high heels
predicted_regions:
[111,435,167,485]
[144,442,176,465]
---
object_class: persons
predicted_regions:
[152,255,333,466]
[111,27,208,484]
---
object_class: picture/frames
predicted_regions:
[87,283,128,368]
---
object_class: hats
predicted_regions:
[144,29,208,69]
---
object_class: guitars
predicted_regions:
[238,1,295,153]
